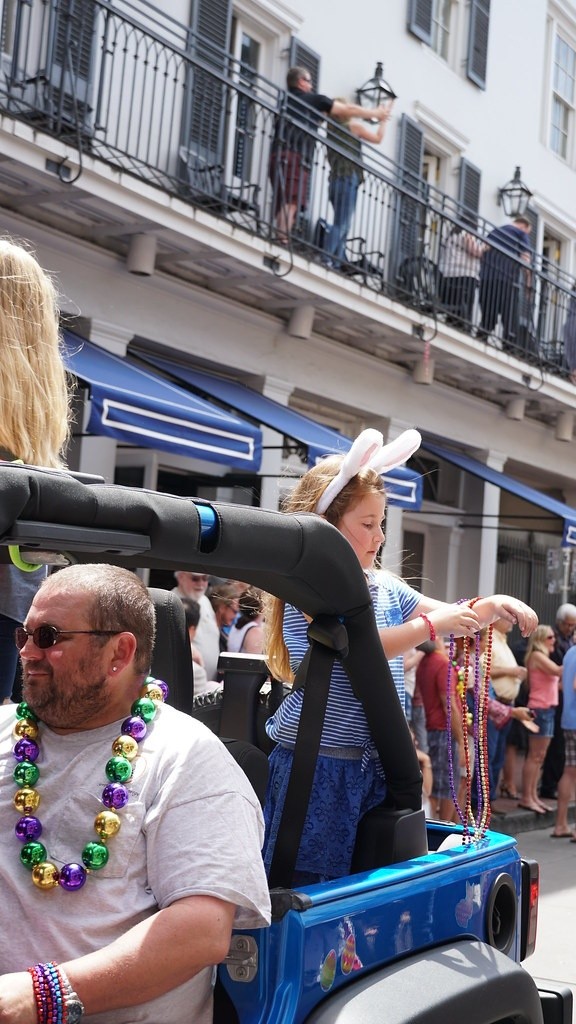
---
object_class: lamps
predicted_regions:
[497,166,532,217]
[287,304,315,340]
[553,410,576,442]
[411,360,434,384]
[128,232,157,276]
[506,400,526,420]
[356,61,396,125]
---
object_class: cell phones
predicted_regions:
[527,710,536,719]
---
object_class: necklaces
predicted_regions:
[447,597,493,845]
[13,677,168,893]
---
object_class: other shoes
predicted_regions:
[518,800,546,816]
[490,800,508,815]
[535,801,553,812]
[538,789,558,801]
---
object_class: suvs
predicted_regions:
[0,460,574,1024]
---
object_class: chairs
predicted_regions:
[402,257,468,331]
[146,585,271,818]
[6,71,95,154]
[318,218,384,292]
[520,325,565,374]
[179,146,260,233]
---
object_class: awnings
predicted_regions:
[420,446,576,547]
[130,348,422,507]
[59,330,263,472]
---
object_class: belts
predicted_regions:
[495,696,515,705]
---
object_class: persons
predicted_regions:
[270,69,392,270]
[466,630,531,826]
[411,636,462,823]
[403,649,424,719]
[440,216,535,350]
[168,571,268,693]
[0,237,66,708]
[563,285,576,385]
[260,456,539,885]
[0,564,272,1024]
[481,603,576,845]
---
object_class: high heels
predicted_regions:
[498,780,521,801]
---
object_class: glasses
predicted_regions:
[12,625,140,653]
[545,634,556,640]
[302,76,314,85]
[225,603,239,617]
[184,573,211,582]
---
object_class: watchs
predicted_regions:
[57,965,84,1024]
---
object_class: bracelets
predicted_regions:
[418,613,435,640]
[28,961,68,1024]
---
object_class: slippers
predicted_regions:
[570,837,576,843]
[550,827,576,837]
